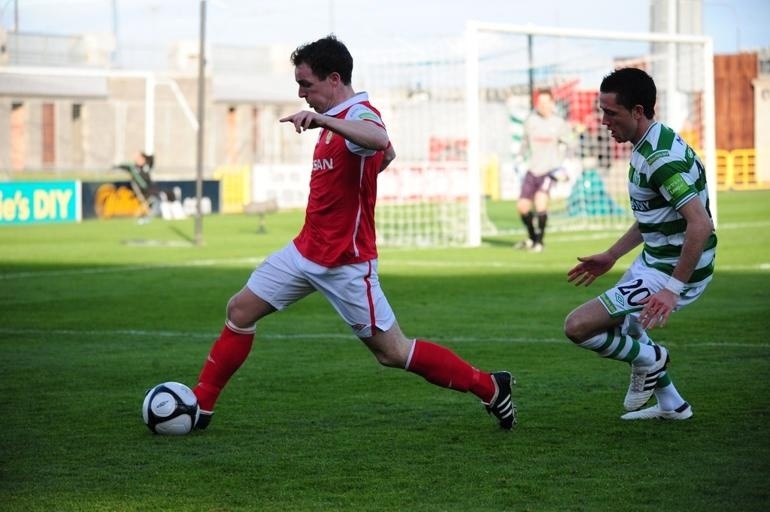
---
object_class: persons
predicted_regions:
[175,35,521,438]
[560,64,720,421]
[113,154,175,215]
[517,88,571,254]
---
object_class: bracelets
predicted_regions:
[665,277,683,295]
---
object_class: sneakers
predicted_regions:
[620,344,669,411]
[480,371,518,432]
[620,405,692,421]
[198,409,215,432]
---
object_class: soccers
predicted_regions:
[143,382,199,436]
